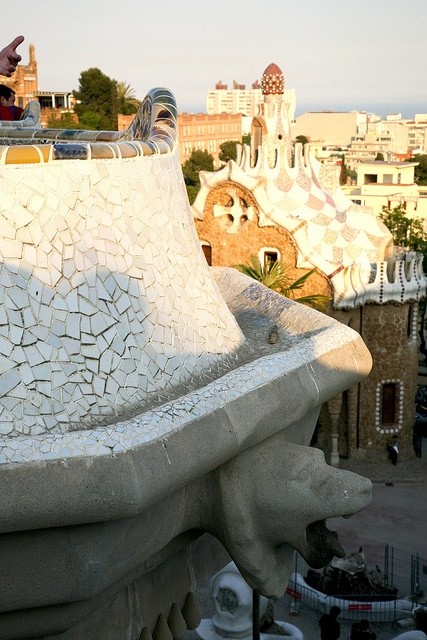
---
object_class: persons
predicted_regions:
[351,619,376,639]
[0,84,26,120]
[384,434,400,486]
[319,605,343,637]
[0,35,25,77]
